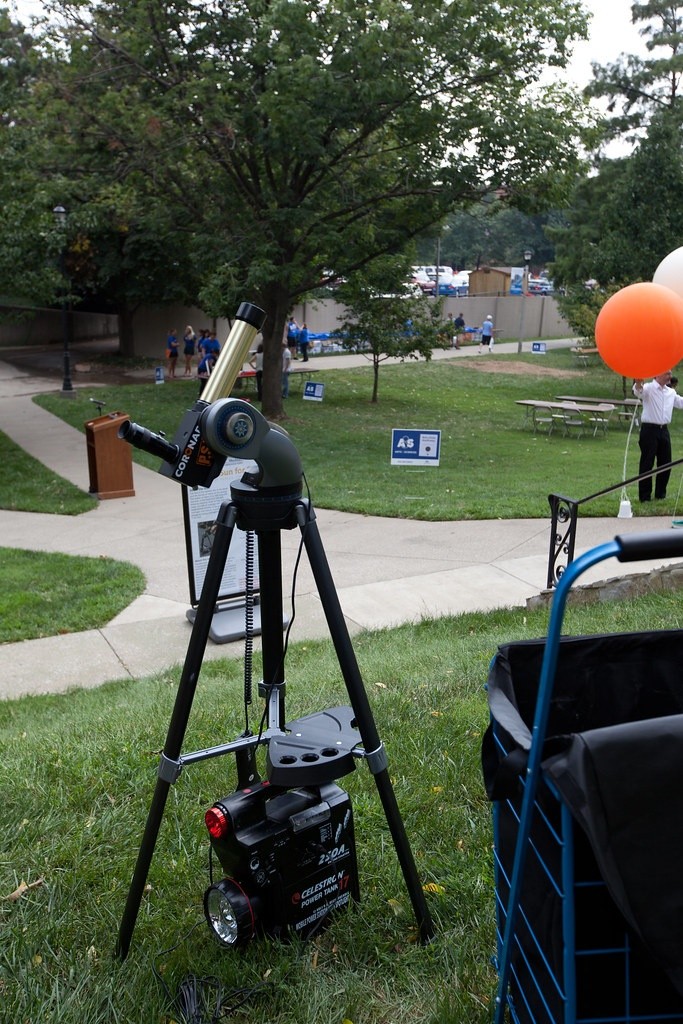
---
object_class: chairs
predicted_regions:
[532,398,641,440]
[574,347,590,368]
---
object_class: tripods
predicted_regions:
[107,479,435,981]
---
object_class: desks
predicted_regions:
[195,368,320,401]
[515,400,618,439]
[555,396,642,432]
[570,348,599,369]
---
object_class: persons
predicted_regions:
[631,371,683,502]
[166,318,309,401]
[198,522,218,556]
[478,315,494,354]
[445,313,467,349]
[666,377,679,388]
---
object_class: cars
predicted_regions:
[528,271,565,296]
[322,266,473,298]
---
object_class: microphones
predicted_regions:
[89,398,106,405]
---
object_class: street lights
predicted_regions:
[53,206,73,391]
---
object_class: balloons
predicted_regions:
[653,246,683,296]
[595,282,683,379]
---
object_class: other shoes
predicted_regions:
[282,394,288,399]
